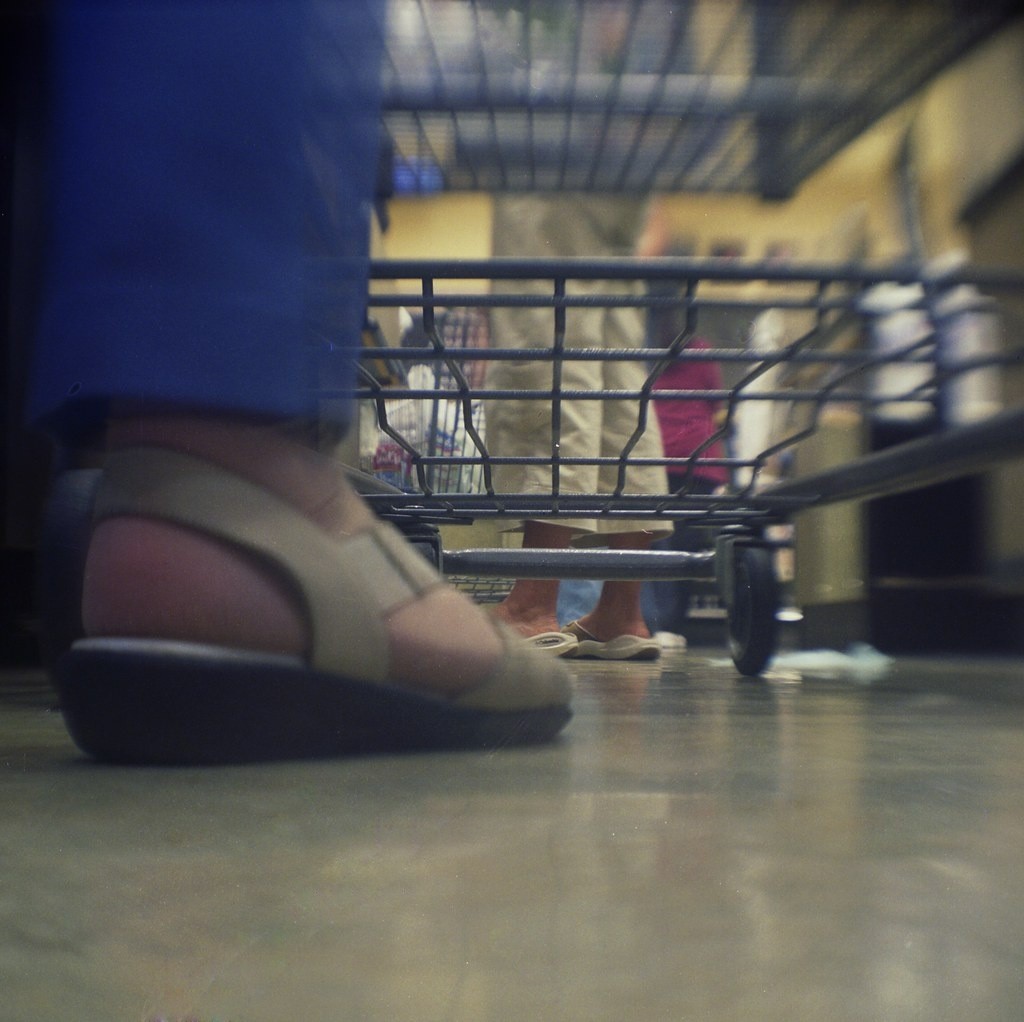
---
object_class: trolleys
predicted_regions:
[350,0,1024,673]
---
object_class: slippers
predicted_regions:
[521,630,579,658]
[561,620,662,660]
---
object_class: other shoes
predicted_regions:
[653,631,688,647]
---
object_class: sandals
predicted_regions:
[59,447,574,762]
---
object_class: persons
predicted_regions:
[457,1,787,659]
[858,173,998,656]
[0,0,576,760]
